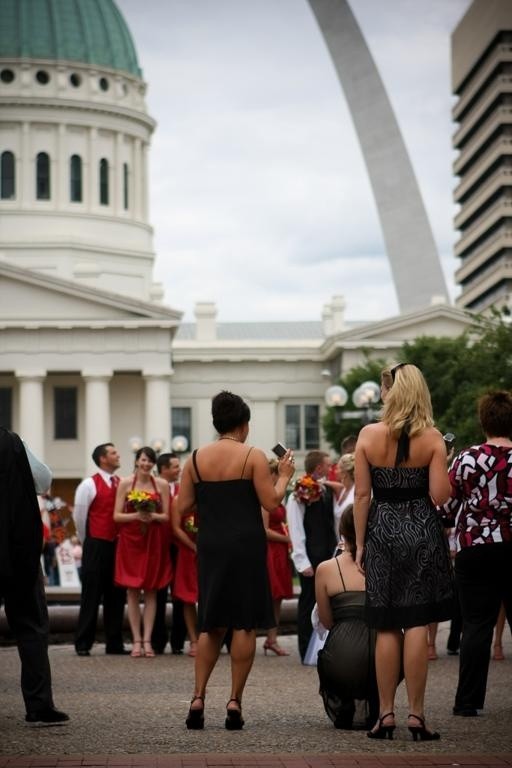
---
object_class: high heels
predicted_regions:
[407,714,440,741]
[367,712,396,740]
[263,640,290,656]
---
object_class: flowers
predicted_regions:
[184,514,199,534]
[127,489,160,537]
[293,472,322,503]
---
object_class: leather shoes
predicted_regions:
[106,648,131,655]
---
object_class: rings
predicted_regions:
[288,456,293,462]
[292,460,295,463]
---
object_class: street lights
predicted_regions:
[129,433,189,471]
[324,378,386,428]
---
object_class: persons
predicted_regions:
[262,450,373,665]
[444,389,512,718]
[73,443,199,657]
[312,503,408,732]
[176,390,296,731]
[427,436,507,663]
[1,423,74,729]
[350,362,463,744]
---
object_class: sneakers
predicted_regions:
[24,709,70,726]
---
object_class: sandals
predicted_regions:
[131,640,155,657]
[186,696,204,730]
[225,699,244,729]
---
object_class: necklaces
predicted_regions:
[219,436,240,442]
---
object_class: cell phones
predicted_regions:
[271,442,288,458]
